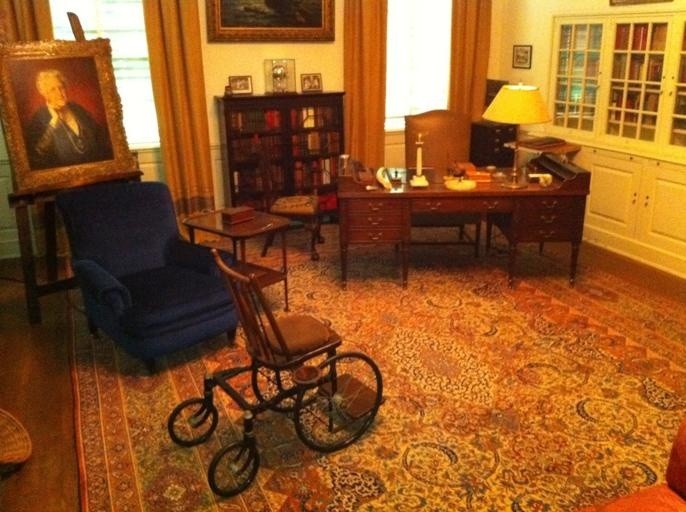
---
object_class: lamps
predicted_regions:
[481,85,552,190]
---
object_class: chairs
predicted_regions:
[56,181,239,375]
[395,109,481,259]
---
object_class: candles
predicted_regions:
[415,133,424,177]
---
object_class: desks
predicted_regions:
[335,152,591,290]
[504,138,581,156]
[183,206,290,312]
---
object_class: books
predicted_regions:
[518,136,567,149]
[555,24,666,124]
[230,107,342,213]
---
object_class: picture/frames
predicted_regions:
[512,45,532,69]
[301,74,323,93]
[229,76,252,95]
[0,37,144,198]
[205,0,335,44]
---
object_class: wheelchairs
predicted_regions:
[169,247,383,497]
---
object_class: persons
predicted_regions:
[27,67,105,171]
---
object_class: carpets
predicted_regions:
[66,221,686,511]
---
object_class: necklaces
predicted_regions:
[58,117,86,155]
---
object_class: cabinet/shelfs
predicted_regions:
[469,122,517,168]
[8,170,143,324]
[546,8,686,280]
[213,90,346,225]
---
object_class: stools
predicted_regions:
[261,195,325,261]
